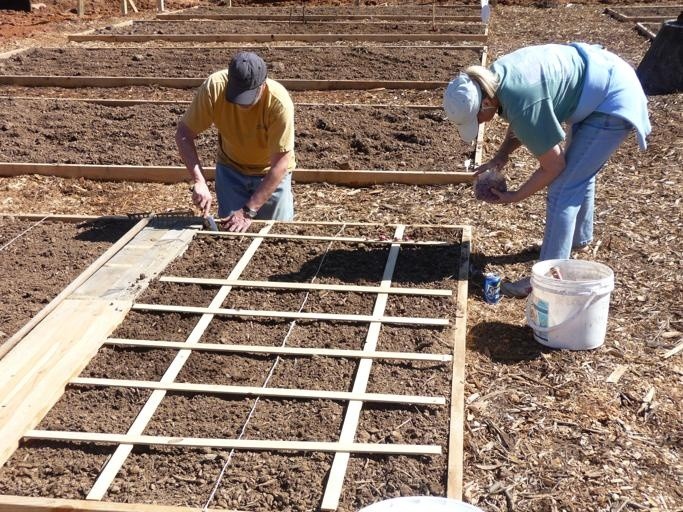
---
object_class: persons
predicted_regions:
[441,40,650,299]
[173,52,296,233]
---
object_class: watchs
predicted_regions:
[242,205,257,218]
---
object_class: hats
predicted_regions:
[223,52,269,106]
[443,72,485,143]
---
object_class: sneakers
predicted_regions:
[498,274,533,300]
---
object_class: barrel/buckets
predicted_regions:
[525,259,614,349]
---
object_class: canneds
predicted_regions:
[484,274,501,304]
